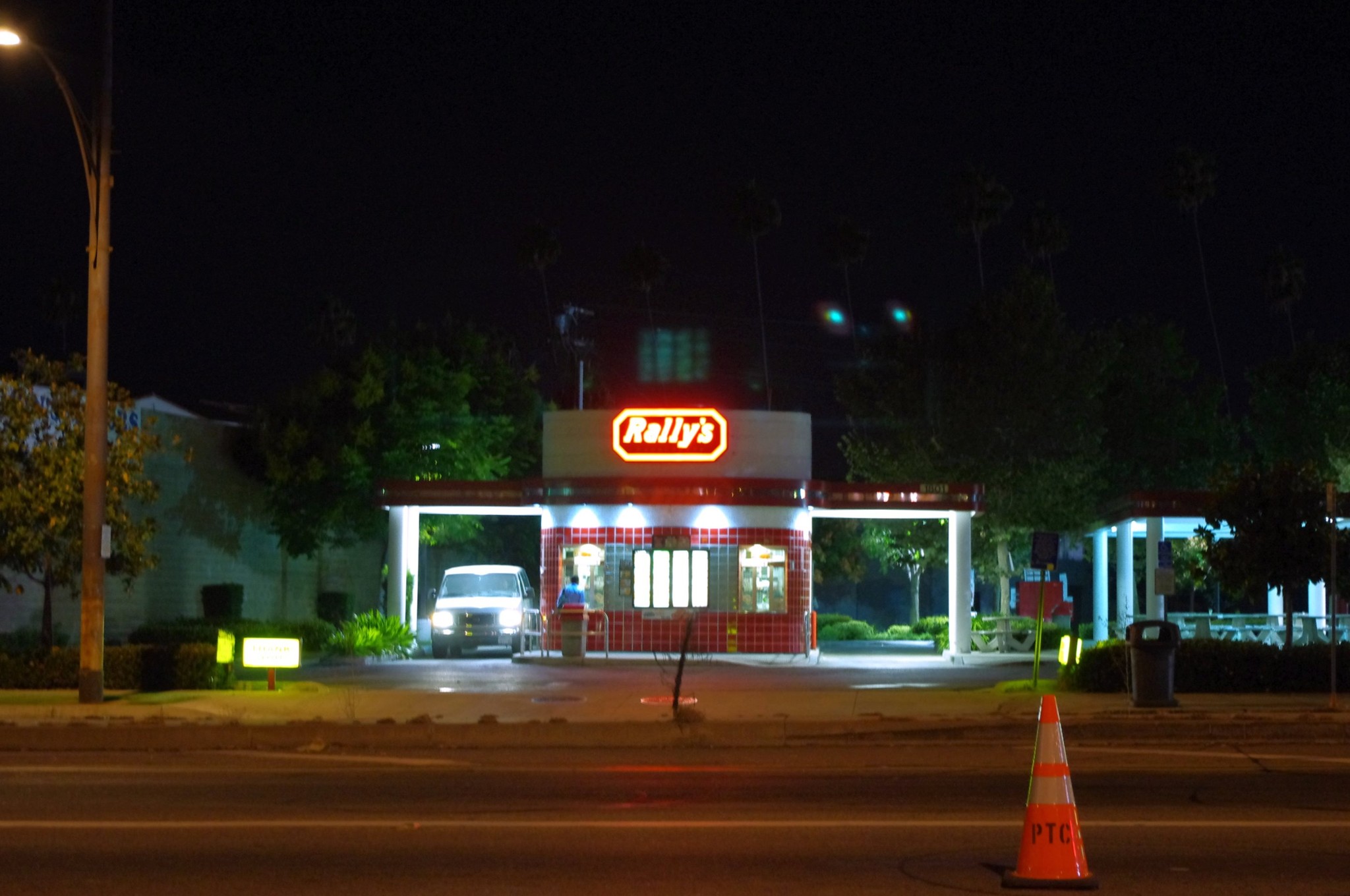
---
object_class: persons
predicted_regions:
[557,575,586,619]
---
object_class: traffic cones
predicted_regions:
[999,693,1100,891]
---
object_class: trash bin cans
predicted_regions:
[1124,619,1181,707]
[559,603,591,657]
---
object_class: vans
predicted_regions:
[426,562,538,660]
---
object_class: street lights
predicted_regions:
[0,20,118,707]
